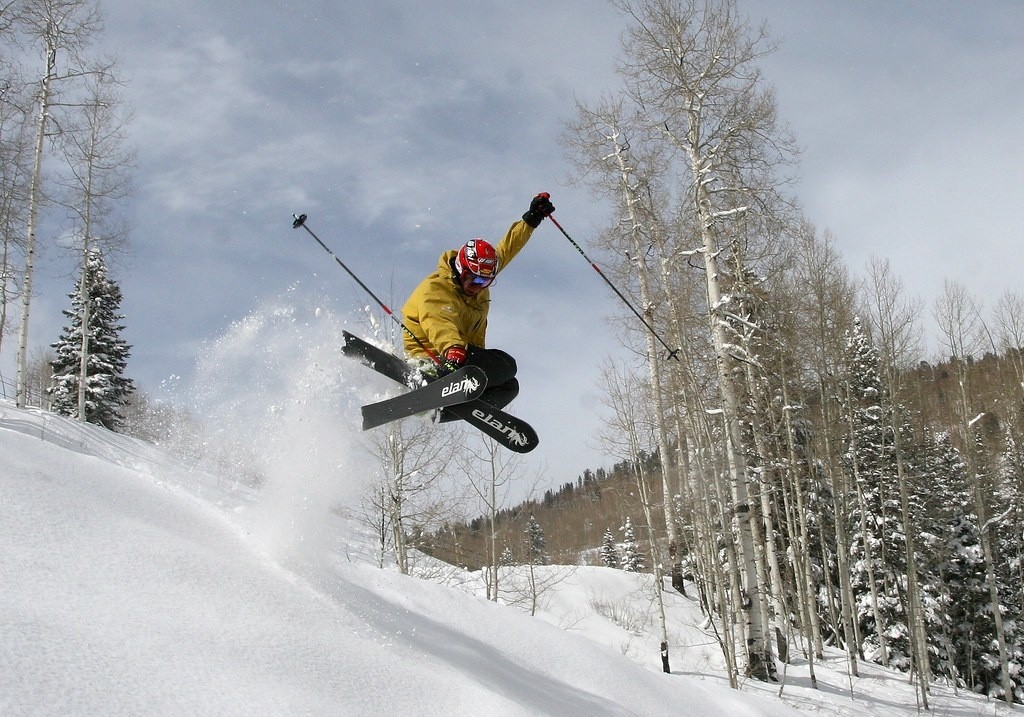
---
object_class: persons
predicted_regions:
[401,192,556,423]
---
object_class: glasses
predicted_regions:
[474,277,492,287]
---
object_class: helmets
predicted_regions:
[455,239,499,289]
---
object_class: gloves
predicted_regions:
[522,192,555,228]
[444,347,467,373]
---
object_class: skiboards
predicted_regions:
[338,329,541,454]
